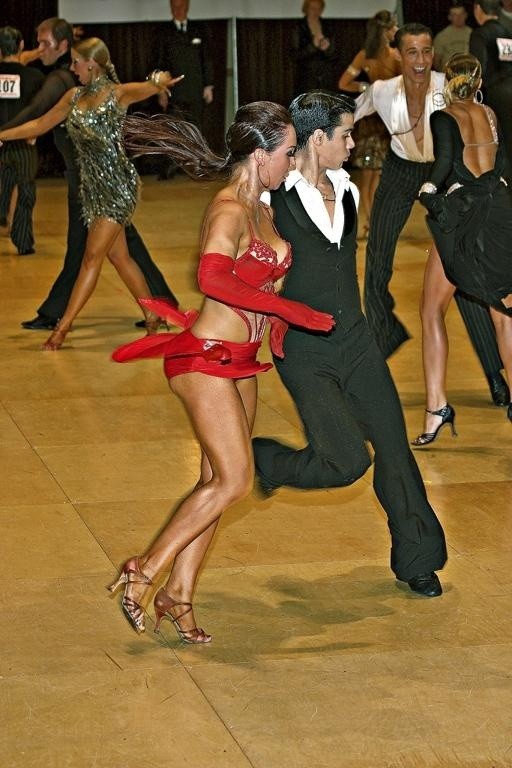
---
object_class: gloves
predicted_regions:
[266,314,288,359]
[418,183,438,199]
[195,253,337,335]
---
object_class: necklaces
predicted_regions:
[315,185,334,201]
[79,75,110,97]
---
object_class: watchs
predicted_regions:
[362,84,366,93]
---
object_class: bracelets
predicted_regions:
[146,68,163,86]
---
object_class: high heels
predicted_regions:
[108,556,155,635]
[143,316,171,336]
[150,587,211,646]
[412,402,457,447]
[43,325,70,350]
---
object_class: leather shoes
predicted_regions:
[408,571,443,597]
[134,319,146,328]
[485,370,511,408]
[249,474,275,502]
[20,315,58,330]
[23,248,34,254]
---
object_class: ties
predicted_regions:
[180,23,184,31]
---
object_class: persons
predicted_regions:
[411,52,512,447]
[105,101,334,642]
[0,27,45,256]
[337,11,403,240]
[469,0,512,150]
[19,26,83,66]
[290,0,342,91]
[250,93,447,598]
[155,0,214,182]
[354,23,512,406]
[432,3,471,70]
[497,0,512,36]
